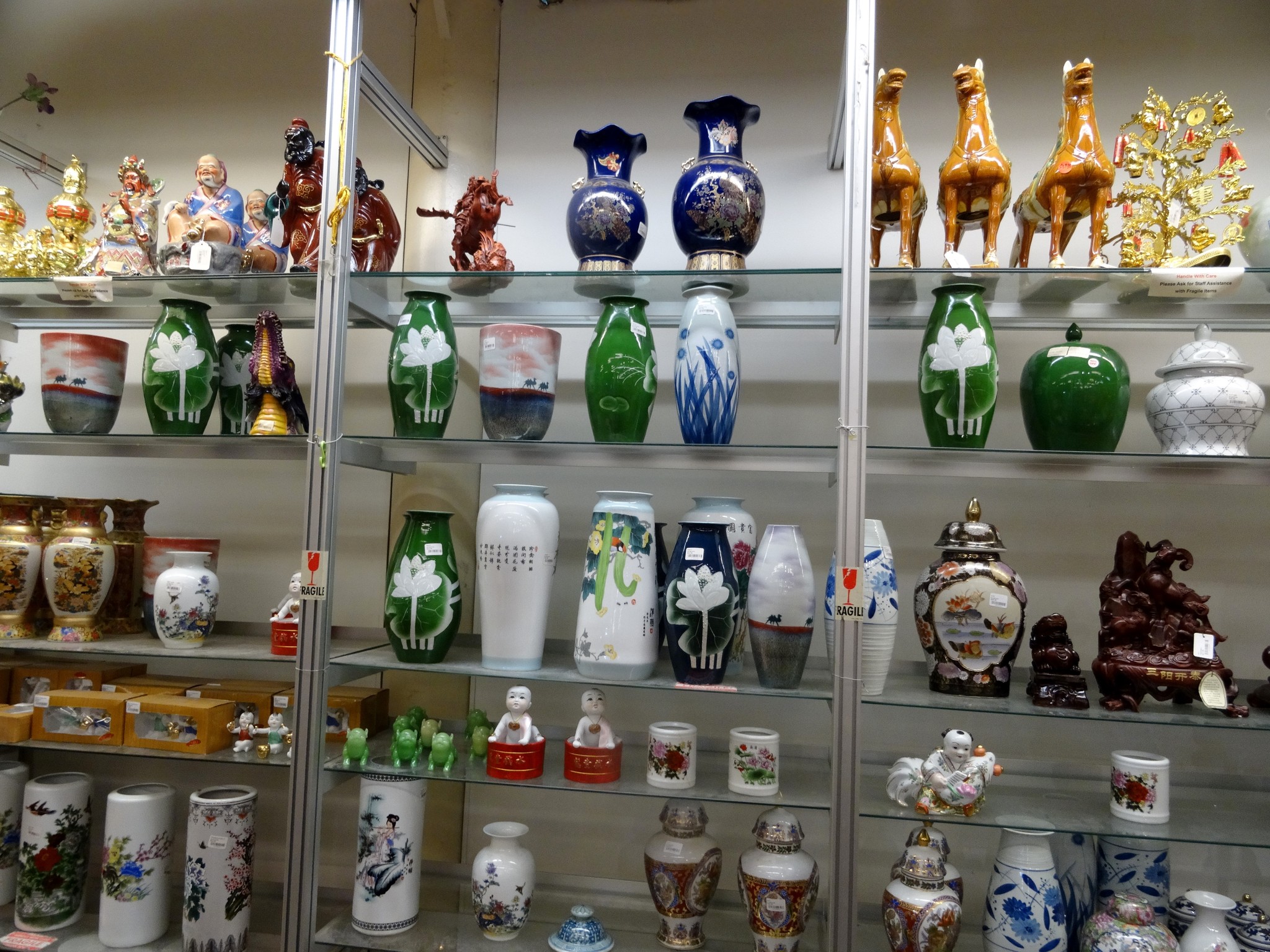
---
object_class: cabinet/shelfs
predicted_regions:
[0,251,1268,950]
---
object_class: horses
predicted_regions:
[871,58,1116,267]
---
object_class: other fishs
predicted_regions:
[885,746,1004,807]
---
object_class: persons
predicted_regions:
[915,728,974,814]
[453,175,493,240]
[227,707,293,760]
[0,118,402,302]
[268,572,304,624]
[484,683,544,745]
[571,688,621,750]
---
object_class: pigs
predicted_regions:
[342,705,493,771]
